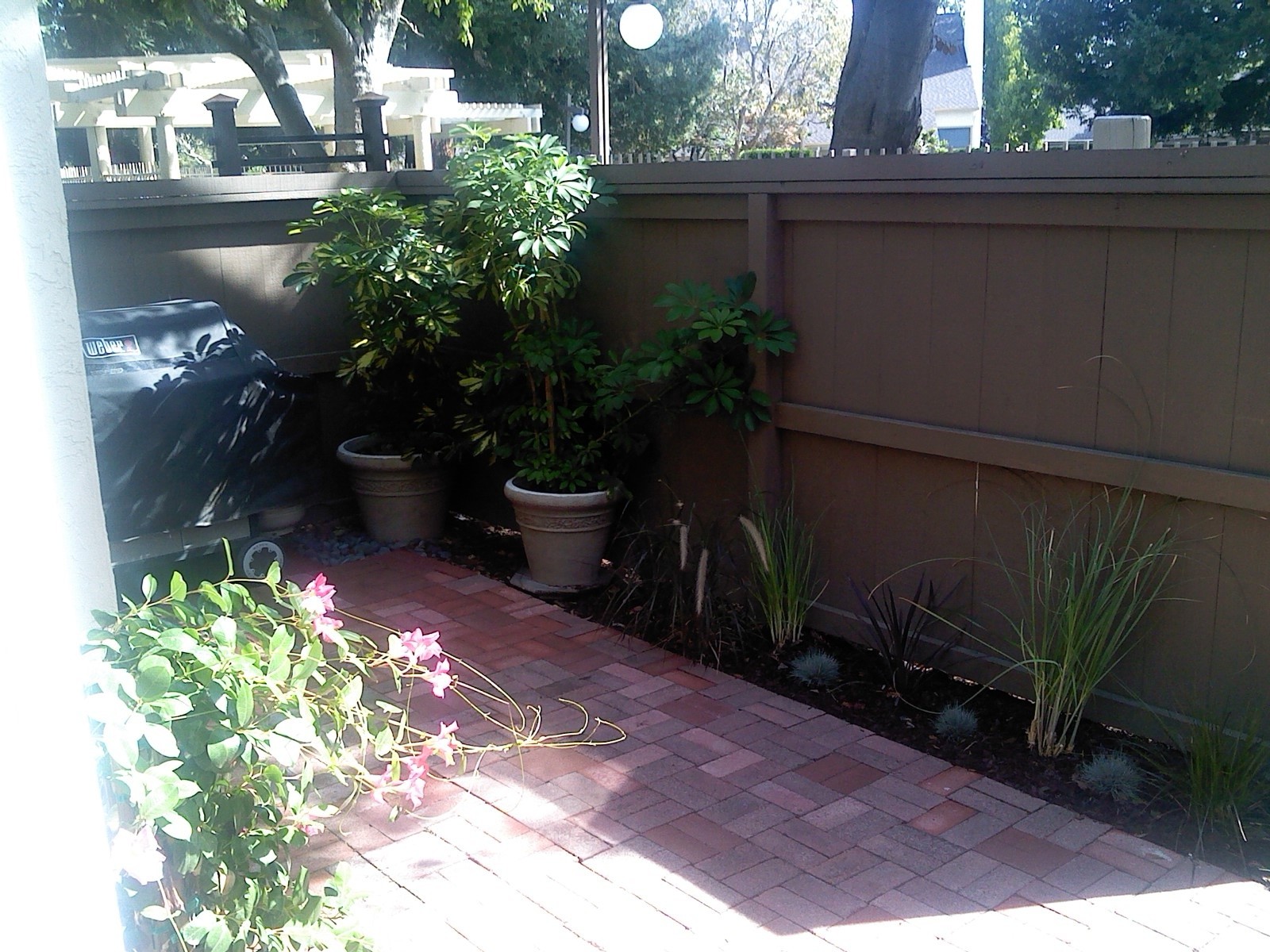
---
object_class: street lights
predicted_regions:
[562,92,590,156]
[587,0,663,163]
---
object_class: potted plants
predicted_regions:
[282,116,800,586]
